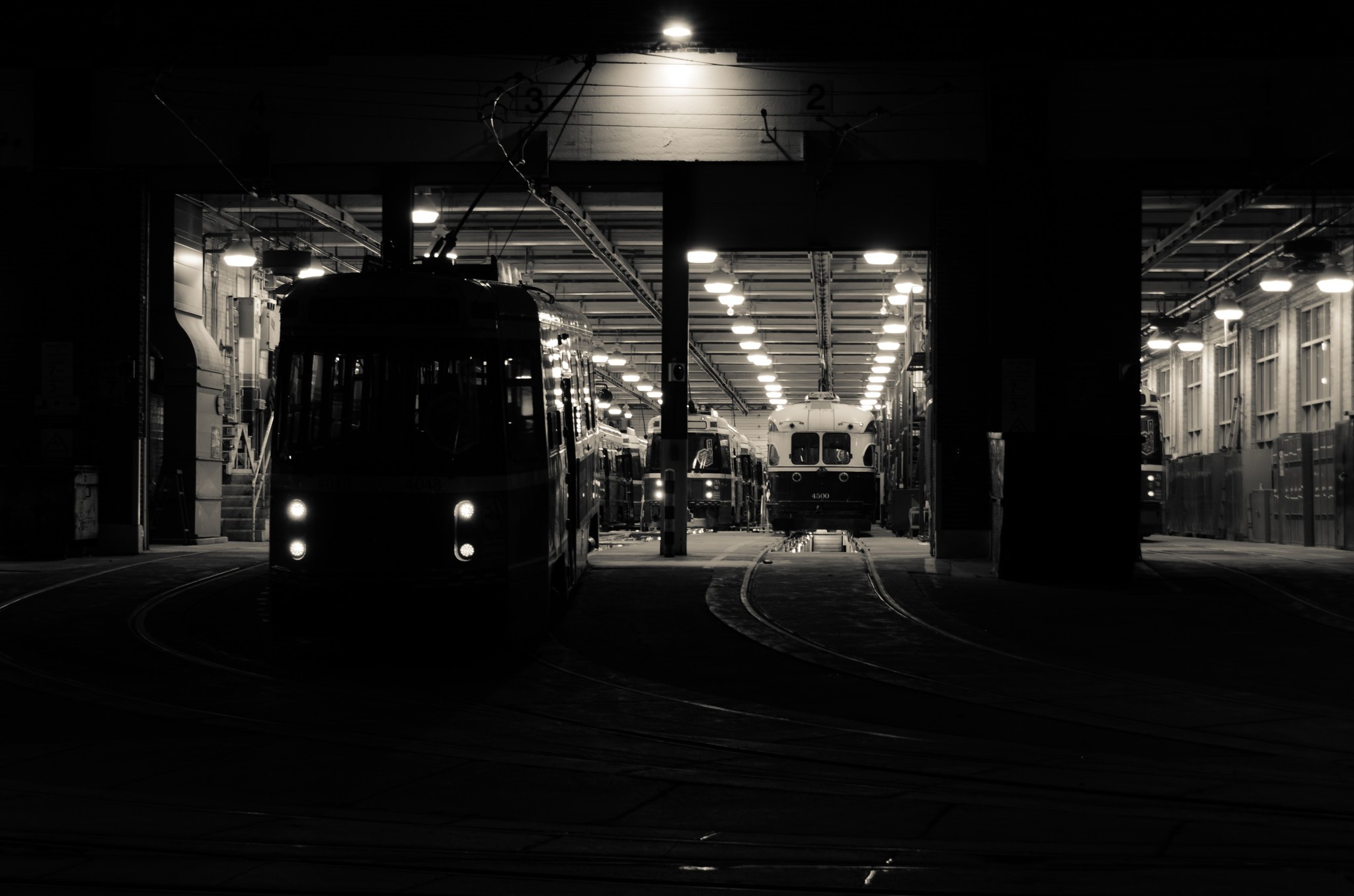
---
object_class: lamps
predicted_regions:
[217,189,1298,410]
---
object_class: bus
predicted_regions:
[764,399,883,536]
[1139,386,1164,536]
[598,419,646,527]
[642,412,765,533]
[254,254,604,604]
